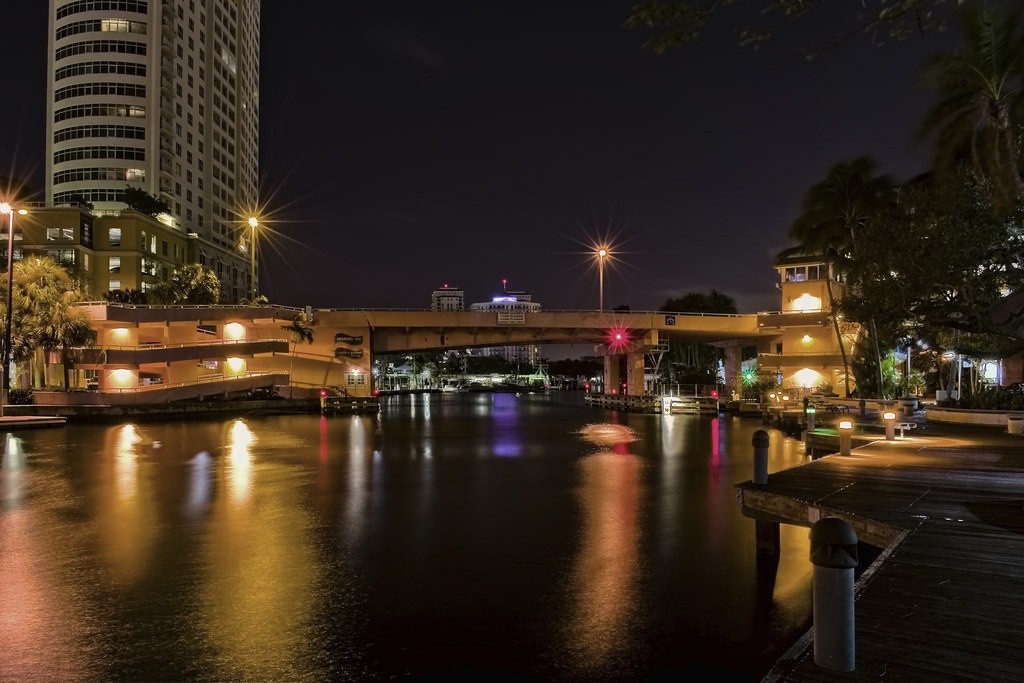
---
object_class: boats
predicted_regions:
[441,378,472,393]
[492,364,540,393]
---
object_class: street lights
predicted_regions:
[597,249,607,313]
[0,200,30,405]
[248,215,259,301]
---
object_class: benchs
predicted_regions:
[830,404,849,417]
